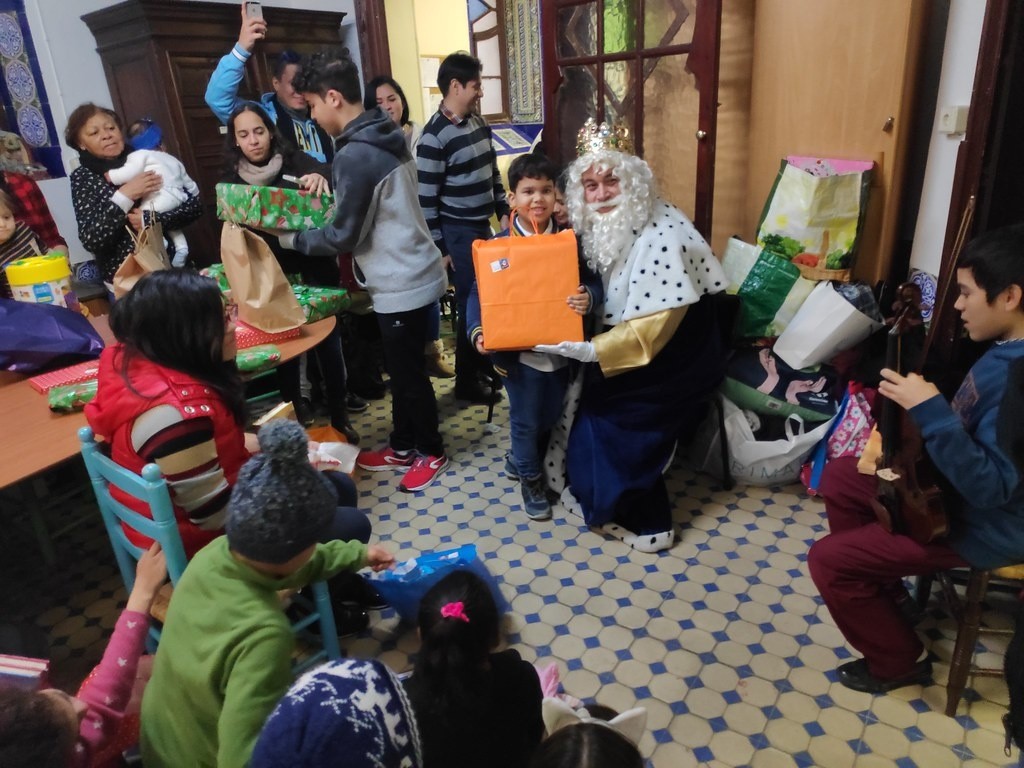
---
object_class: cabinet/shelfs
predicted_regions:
[81,0,347,270]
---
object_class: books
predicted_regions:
[0,653,49,671]
[0,665,47,694]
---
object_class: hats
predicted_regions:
[227,418,338,563]
[247,658,423,768]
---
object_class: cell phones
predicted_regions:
[245,2,264,36]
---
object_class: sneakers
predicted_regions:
[311,381,369,410]
[356,445,421,474]
[519,472,552,519]
[398,453,450,491]
[504,453,520,478]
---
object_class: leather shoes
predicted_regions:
[896,595,922,625]
[837,654,933,692]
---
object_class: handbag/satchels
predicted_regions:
[472,206,584,351]
[677,154,887,498]
[220,198,308,334]
[113,200,172,302]
[379,543,508,625]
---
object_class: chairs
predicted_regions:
[79,427,342,685]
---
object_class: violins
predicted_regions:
[871,282,951,545]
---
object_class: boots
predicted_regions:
[424,339,456,378]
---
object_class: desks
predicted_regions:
[0,293,337,607]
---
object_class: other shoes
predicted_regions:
[341,573,388,610]
[456,378,503,405]
[332,416,359,444]
[307,610,368,639]
[297,397,313,425]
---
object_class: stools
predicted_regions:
[913,562,1024,718]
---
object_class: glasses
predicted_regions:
[222,303,238,326]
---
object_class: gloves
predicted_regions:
[530,341,599,363]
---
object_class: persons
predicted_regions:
[0,260,653,768]
[0,0,727,549]
[805,219,1024,693]
